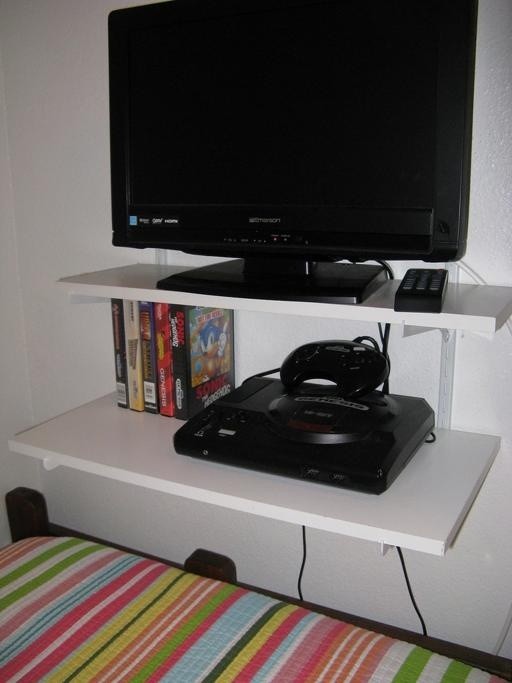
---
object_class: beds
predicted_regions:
[0,486,512,683]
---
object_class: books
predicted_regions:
[153,303,175,417]
[110,299,130,410]
[123,300,144,411]
[139,302,159,413]
[171,304,235,420]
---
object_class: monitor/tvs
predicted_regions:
[108,1,479,302]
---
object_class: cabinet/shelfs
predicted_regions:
[6,264,512,556]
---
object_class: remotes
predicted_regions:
[394,269,448,313]
